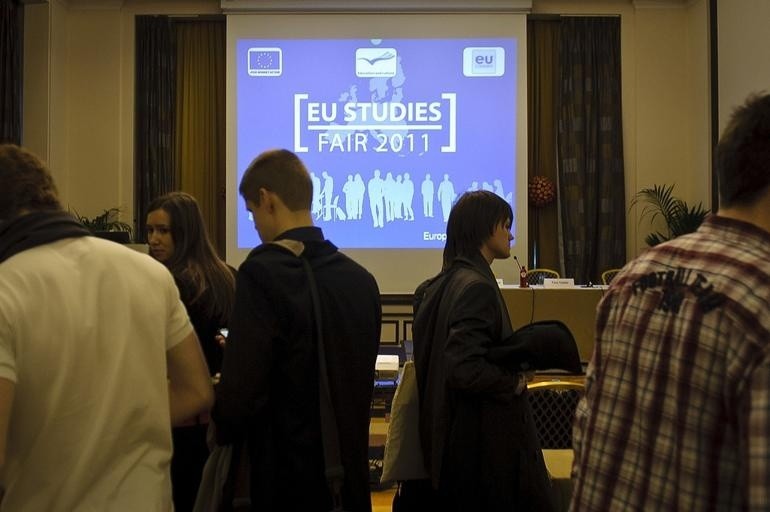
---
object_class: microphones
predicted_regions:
[513,255,531,290]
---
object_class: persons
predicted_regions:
[144,191,237,512]
[210,148,386,512]
[310,166,513,229]
[560,89,770,512]
[0,143,218,512]
[410,188,562,512]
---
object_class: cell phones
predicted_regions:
[217,326,228,340]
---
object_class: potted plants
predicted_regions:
[87,209,128,241]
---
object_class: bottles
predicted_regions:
[520,265,527,288]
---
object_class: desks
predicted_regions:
[495,284,610,365]
[541,448,574,501]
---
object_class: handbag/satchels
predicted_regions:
[376,355,428,485]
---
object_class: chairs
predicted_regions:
[525,268,623,286]
[506,317,595,450]
